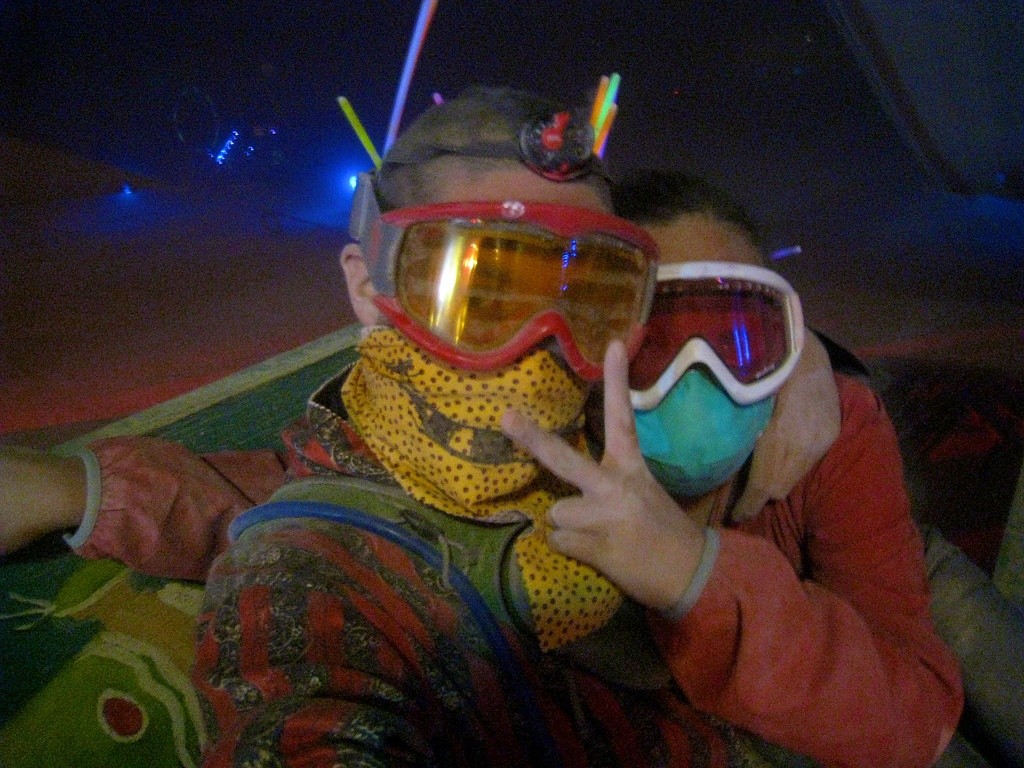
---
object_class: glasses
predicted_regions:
[626,261,802,411]
[364,200,660,384]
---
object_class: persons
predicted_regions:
[0,78,1024,768]
